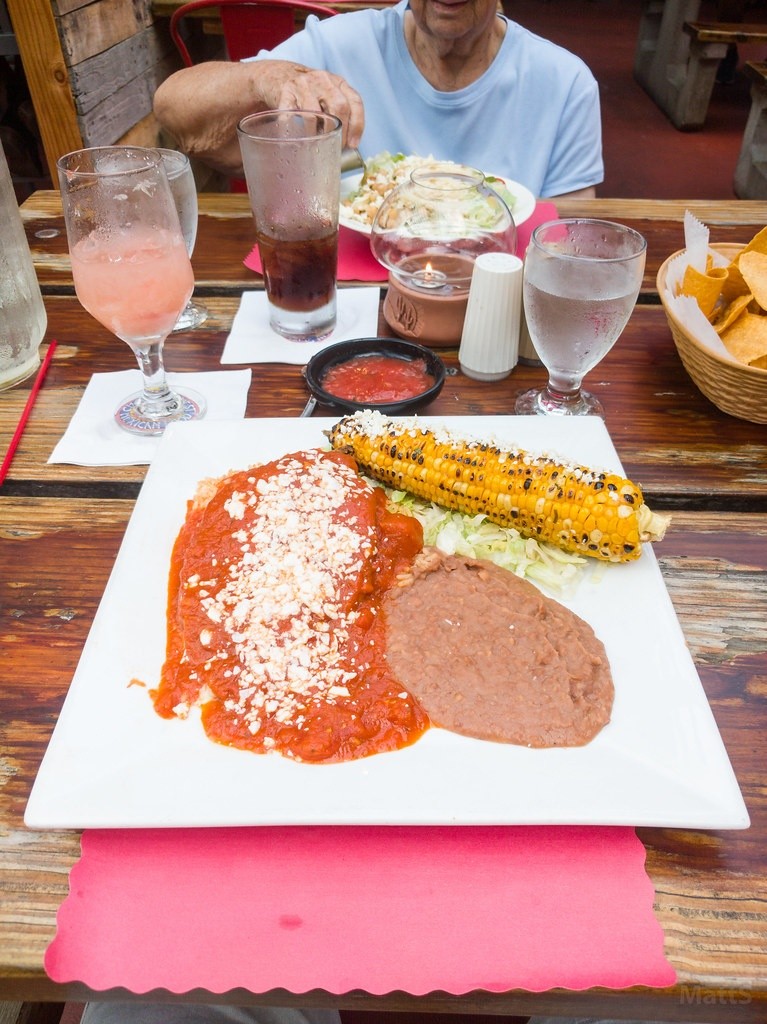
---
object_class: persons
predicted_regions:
[153,0,604,199]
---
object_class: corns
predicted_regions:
[327,413,674,564]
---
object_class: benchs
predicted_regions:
[674,21,767,134]
[732,59,767,200]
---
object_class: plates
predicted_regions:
[306,337,446,416]
[21,415,751,831]
[338,162,536,244]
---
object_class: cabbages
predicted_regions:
[379,488,591,592]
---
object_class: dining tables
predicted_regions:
[0,192,767,1024]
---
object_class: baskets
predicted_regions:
[657,242,767,423]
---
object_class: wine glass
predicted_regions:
[513,218,648,423]
[55,144,208,438]
[92,147,207,336]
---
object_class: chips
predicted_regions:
[677,223,766,372]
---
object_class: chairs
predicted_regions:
[169,0,342,68]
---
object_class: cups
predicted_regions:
[235,108,343,344]
[458,252,524,382]
[0,141,49,392]
[518,242,569,369]
